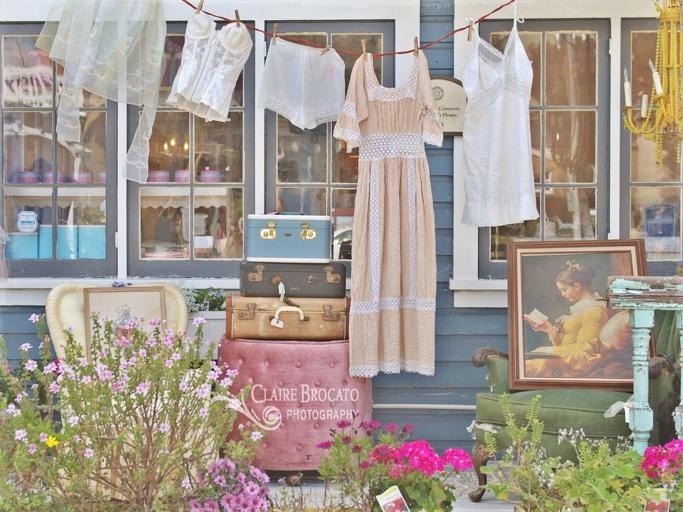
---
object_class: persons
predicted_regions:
[523,262,607,378]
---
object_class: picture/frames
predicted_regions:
[504,235,648,392]
[82,285,168,365]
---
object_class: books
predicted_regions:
[523,308,549,329]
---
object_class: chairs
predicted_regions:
[43,284,189,366]
[470,308,682,505]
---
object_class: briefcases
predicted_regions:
[226,212,350,340]
